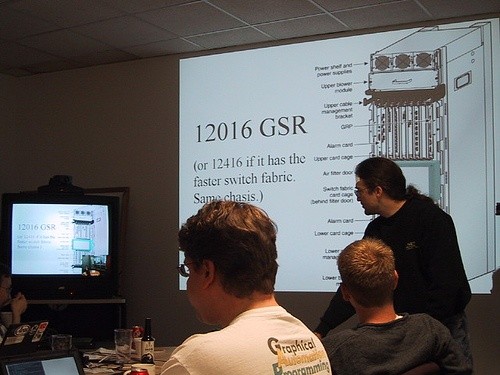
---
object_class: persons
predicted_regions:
[0,263,28,343]
[314,156,472,375]
[165,200,331,375]
[322,238,472,375]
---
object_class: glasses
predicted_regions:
[177,259,221,278]
[355,184,378,196]
[0,286,12,293]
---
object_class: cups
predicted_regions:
[51,334,72,351]
[123,364,156,375]
[114,329,134,364]
[134,338,142,357]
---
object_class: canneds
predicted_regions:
[130,369,149,375]
[130,325,143,350]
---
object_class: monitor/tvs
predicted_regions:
[0,192,120,298]
[0,348,86,375]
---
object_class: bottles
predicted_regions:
[141,317,155,364]
